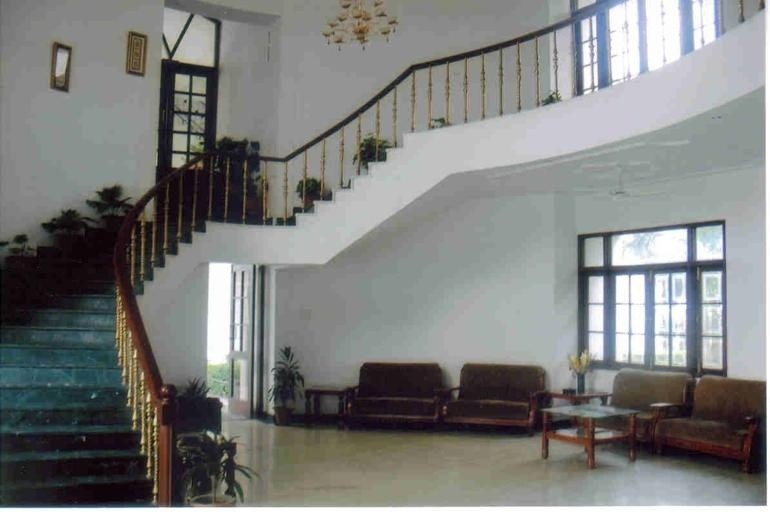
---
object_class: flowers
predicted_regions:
[570,348,593,374]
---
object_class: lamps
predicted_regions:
[322,1,404,53]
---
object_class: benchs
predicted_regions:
[343,360,547,438]
[576,369,765,473]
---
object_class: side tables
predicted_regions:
[307,382,354,431]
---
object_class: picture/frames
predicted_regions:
[50,41,72,91]
[126,30,147,76]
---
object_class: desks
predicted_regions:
[540,386,641,468]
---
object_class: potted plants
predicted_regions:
[0,184,135,305]
[179,430,259,508]
[267,344,304,425]
[296,177,323,208]
[220,135,264,216]
